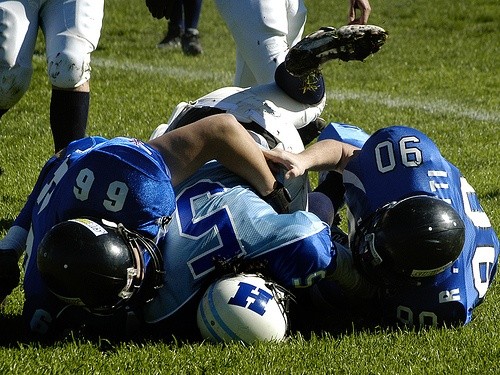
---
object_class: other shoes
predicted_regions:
[182,28,201,55]
[0,249,21,304]
[160,23,183,44]
[284,25,387,77]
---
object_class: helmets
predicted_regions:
[353,194,465,279]
[196,271,296,344]
[35,217,164,315]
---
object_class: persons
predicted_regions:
[213,0,374,87]
[0,25,500,346]
[0,0,102,153]
[146,0,203,56]
